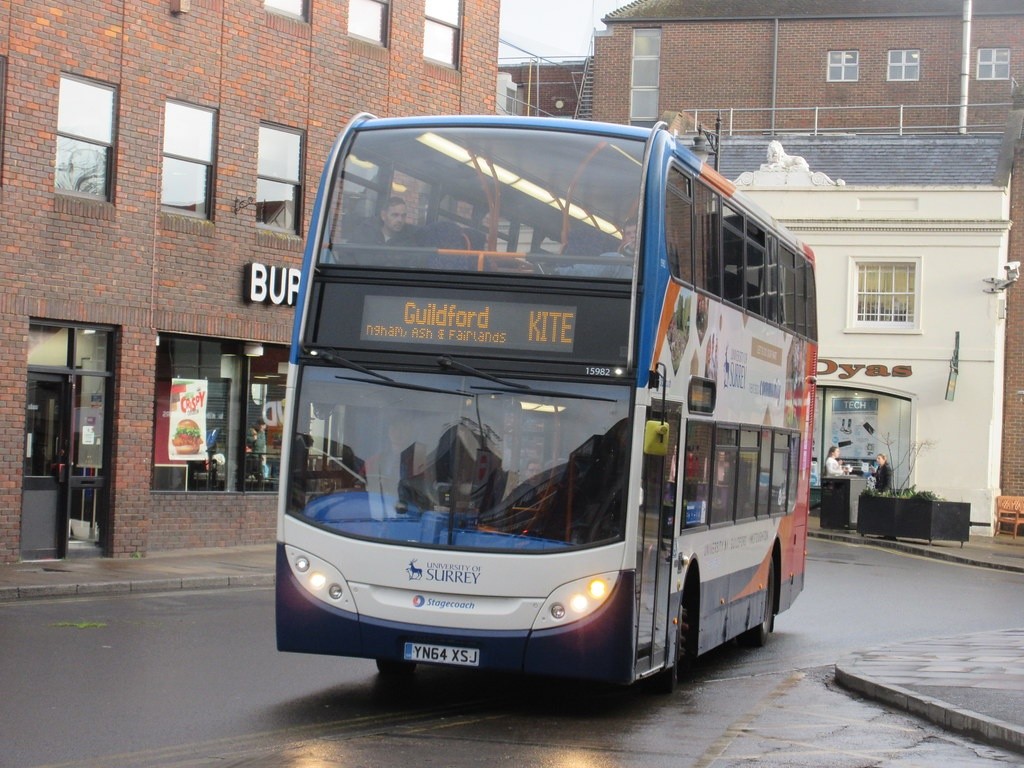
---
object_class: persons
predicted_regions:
[523,458,545,488]
[245,417,267,479]
[354,412,437,508]
[335,196,425,268]
[871,453,892,491]
[824,446,849,477]
[667,444,701,502]
[618,218,639,270]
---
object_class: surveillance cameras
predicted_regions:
[1003,261,1020,271]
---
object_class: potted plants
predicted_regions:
[856,486,971,548]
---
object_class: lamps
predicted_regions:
[234,196,286,224]
[244,342,264,356]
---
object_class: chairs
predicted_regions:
[419,220,485,252]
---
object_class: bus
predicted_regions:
[271,111,819,700]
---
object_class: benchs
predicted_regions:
[993,496,1024,539]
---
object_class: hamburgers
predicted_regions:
[172,418,204,455]
